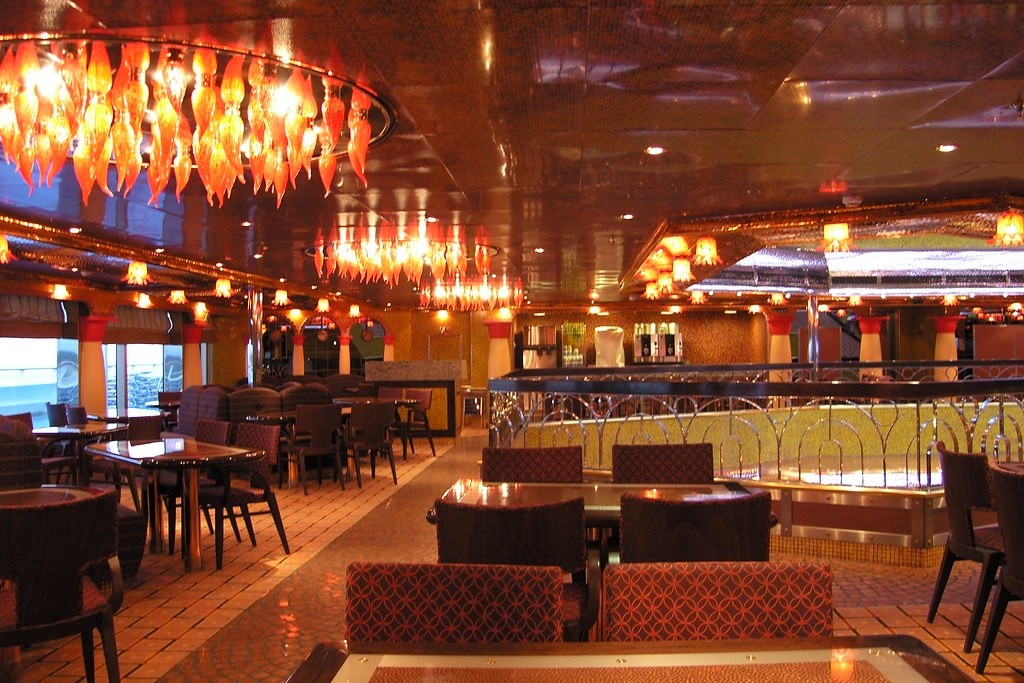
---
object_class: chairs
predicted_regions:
[436,499,603,642]
[610,443,714,485]
[597,559,834,642]
[345,562,564,644]
[604,492,771,566]
[926,441,1024,675]
[481,446,582,483]
[0,375,436,683]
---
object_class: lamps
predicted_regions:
[986,210,1024,250]
[640,237,724,314]
[1,37,373,210]
[817,223,858,255]
[0,234,526,326]
[747,292,1023,313]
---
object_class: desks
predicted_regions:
[85,408,164,446]
[85,438,266,573]
[287,634,976,683]
[245,410,357,490]
[32,423,128,489]
[0,487,105,683]
[426,478,778,565]
[332,397,420,408]
